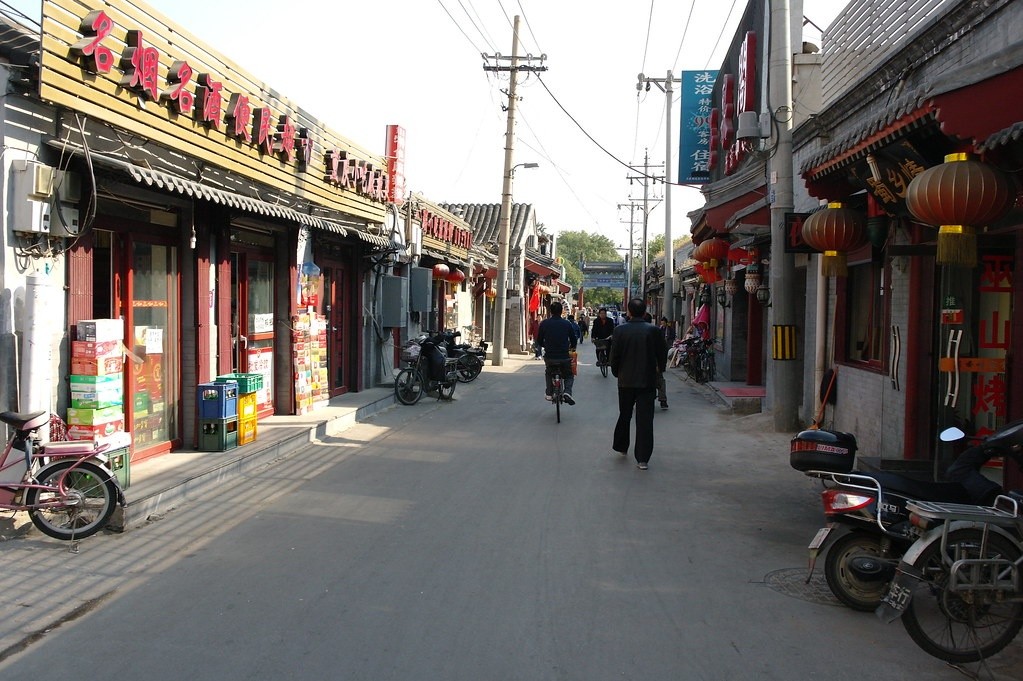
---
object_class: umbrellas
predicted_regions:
[691,303,709,340]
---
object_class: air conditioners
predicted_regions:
[527,234,539,250]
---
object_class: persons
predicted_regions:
[537,302,576,406]
[644,313,668,410]
[660,317,674,372]
[529,314,543,360]
[591,309,614,363]
[567,315,580,352]
[609,298,668,469]
[577,311,590,344]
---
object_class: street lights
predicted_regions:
[491,163,540,366]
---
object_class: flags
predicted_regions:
[529,285,540,312]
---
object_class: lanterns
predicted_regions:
[448,268,464,291]
[432,264,449,287]
[692,237,728,269]
[801,202,867,276]
[906,152,1015,265]
[485,288,496,302]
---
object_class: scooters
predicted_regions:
[435,330,488,364]
[790,422,1023,612]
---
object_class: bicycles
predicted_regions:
[394,332,458,405]
[538,346,576,424]
[676,335,718,384]
[875,491,1022,681]
[592,336,613,378]
[0,410,128,555]
[434,342,482,382]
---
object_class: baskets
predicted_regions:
[401,345,419,362]
[595,339,608,350]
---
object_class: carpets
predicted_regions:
[719,387,766,397]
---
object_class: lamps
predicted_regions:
[680,285,686,301]
[757,280,772,308]
[702,289,712,307]
[717,286,731,309]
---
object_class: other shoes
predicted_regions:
[661,401,669,410]
[636,461,648,470]
[563,393,576,406]
[535,356,542,360]
[544,392,553,401]
[618,452,628,456]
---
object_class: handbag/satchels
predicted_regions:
[584,332,588,340]
[569,352,577,376]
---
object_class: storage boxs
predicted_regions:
[295,315,329,415]
[197,373,263,451]
[56,319,166,498]
[791,429,857,473]
